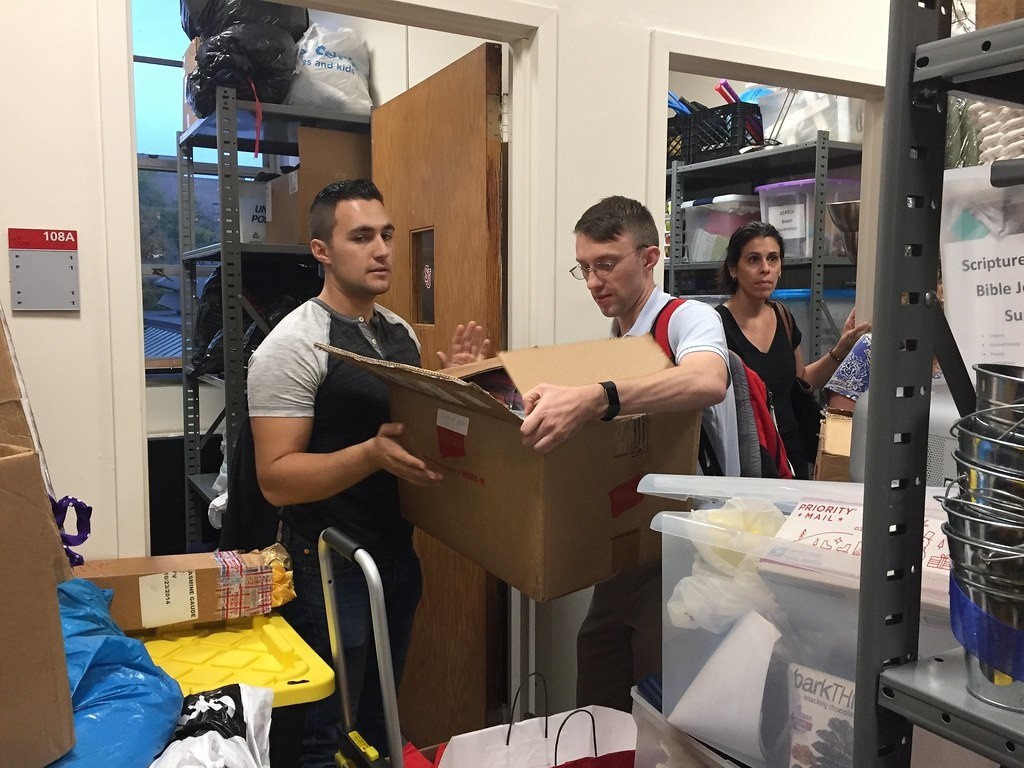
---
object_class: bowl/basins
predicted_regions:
[813,200,861,268]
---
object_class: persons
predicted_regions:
[520,195,742,716]
[714,219,870,479]
[821,258,944,412]
[246,175,491,768]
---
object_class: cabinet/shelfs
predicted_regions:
[183,88,368,557]
[664,131,868,480]
[848,0,1024,768]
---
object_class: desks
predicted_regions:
[131,610,336,710]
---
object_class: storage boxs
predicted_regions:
[635,474,961,768]
[754,178,858,259]
[0,325,275,768]
[264,126,364,245]
[690,102,763,164]
[771,286,853,366]
[678,295,728,308]
[314,332,704,603]
[667,114,690,169]
[681,193,755,263]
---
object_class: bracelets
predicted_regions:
[829,348,843,363]
[598,381,621,422]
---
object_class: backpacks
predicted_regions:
[186,252,322,379]
[650,299,797,479]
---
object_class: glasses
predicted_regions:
[569,243,648,281]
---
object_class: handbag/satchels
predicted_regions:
[279,24,373,116]
[437,673,637,768]
[767,299,824,466]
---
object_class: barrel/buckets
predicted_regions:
[932,363,1024,715]
